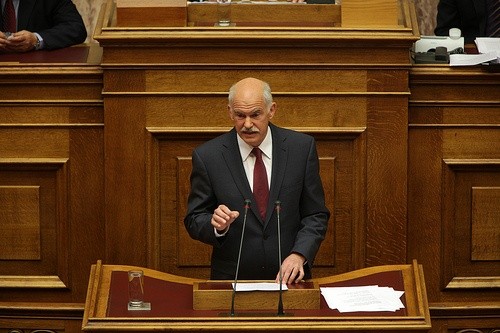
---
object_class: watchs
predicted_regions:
[33,34,41,49]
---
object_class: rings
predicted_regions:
[292,266,300,273]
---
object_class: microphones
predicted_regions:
[230,199,251,313]
[274,200,284,314]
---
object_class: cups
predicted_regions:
[128,269,145,308]
[216,0,231,26]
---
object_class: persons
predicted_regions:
[433,0,500,37]
[183,76,331,285]
[291,0,336,4]
[0,0,87,53]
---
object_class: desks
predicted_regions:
[0,27,500,315]
